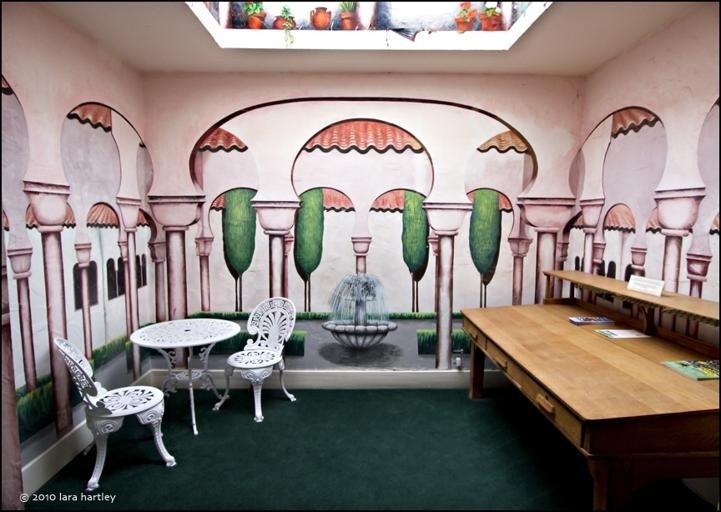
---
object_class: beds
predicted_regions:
[127,318,243,436]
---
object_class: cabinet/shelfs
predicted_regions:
[462,268,720,510]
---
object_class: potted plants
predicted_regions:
[273,8,297,50]
[244,4,267,28]
[339,2,359,30]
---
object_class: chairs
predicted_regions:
[211,295,298,423]
[52,336,180,491]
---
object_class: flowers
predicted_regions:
[451,2,479,24]
[479,2,503,17]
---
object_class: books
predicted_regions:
[592,328,652,340]
[569,316,617,326]
[662,358,721,381]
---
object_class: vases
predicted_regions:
[310,7,333,30]
[456,17,474,31]
[479,13,504,31]
